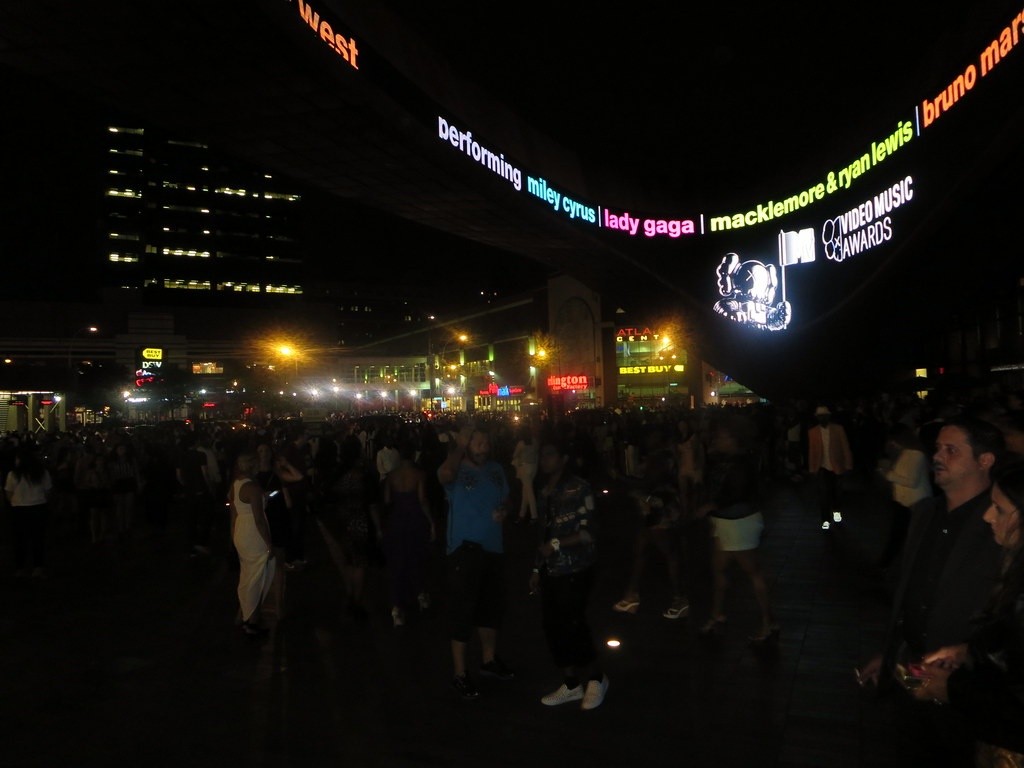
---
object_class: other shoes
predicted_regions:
[833,511,843,523]
[821,521,831,530]
[241,623,264,637]
[866,566,889,576]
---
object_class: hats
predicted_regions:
[814,406,832,417]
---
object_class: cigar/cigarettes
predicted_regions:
[855,668,864,686]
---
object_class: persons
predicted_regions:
[0,385,1024,628]
[526,469,610,709]
[225,454,284,634]
[437,428,515,698]
[919,451,1024,767]
[856,418,1006,768]
[693,416,780,642]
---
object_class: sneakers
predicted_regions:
[451,676,478,696]
[480,659,515,680]
[540,683,585,706]
[581,672,610,710]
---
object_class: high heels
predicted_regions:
[698,616,728,635]
[749,623,781,644]
[613,600,641,615]
[417,594,432,612]
[662,605,689,620]
[391,606,406,628]
